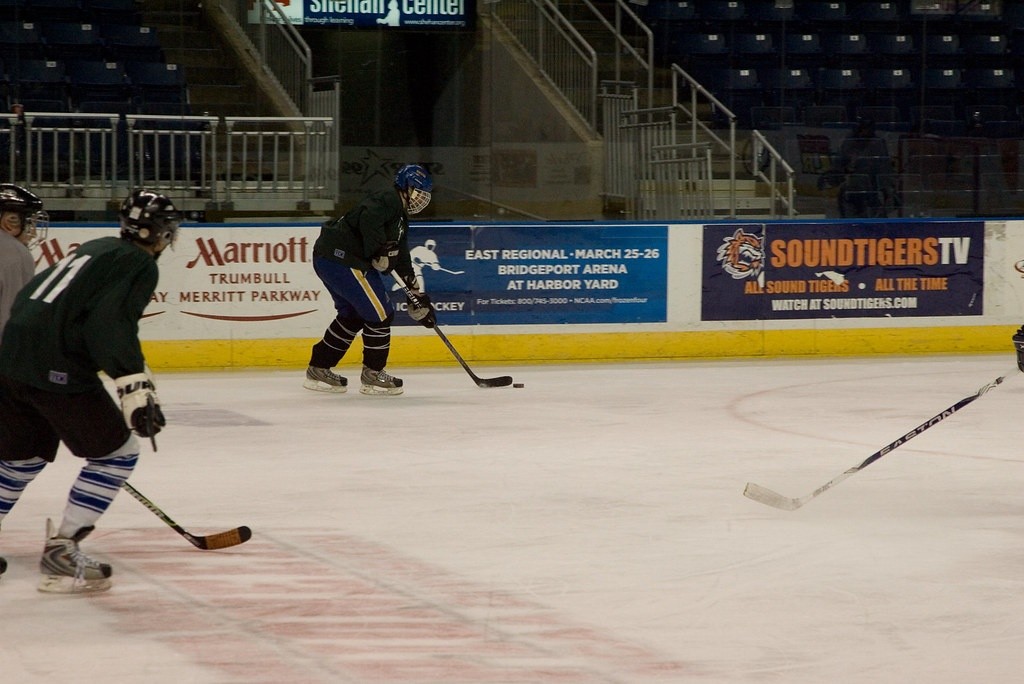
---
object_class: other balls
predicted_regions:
[513,383,524,388]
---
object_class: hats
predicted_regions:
[11,96,24,107]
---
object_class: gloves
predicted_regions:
[405,292,437,328]
[114,362,166,436]
[369,248,395,275]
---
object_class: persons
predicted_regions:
[303,163,437,398]
[0,188,180,593]
[0,184,46,338]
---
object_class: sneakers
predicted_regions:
[39,518,113,579]
[360,363,402,387]
[307,363,348,386]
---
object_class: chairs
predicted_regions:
[620,0,1024,218]
[0,0,201,179]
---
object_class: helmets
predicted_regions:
[393,164,433,215]
[119,187,183,261]
[0,182,50,250]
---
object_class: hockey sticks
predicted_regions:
[122,480,253,552]
[387,265,514,390]
[741,366,1022,512]
